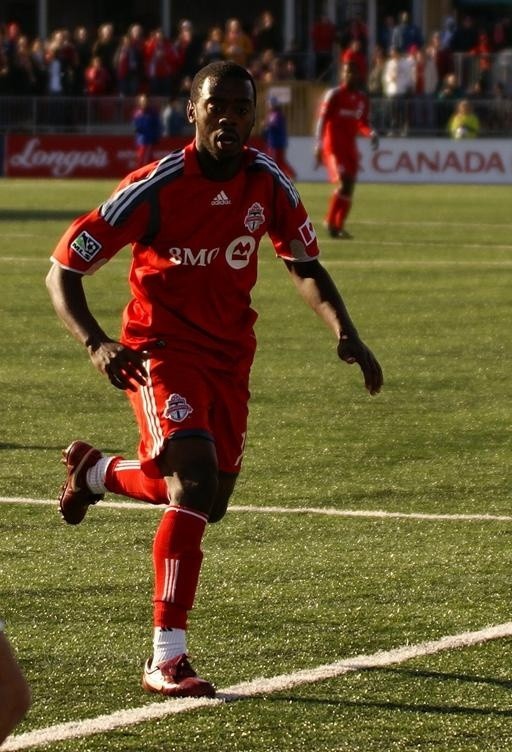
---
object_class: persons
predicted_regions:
[311,63,382,240]
[42,58,388,702]
[0,632,37,749]
[0,8,510,173]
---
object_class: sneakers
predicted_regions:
[143,653,215,699]
[57,440,104,525]
